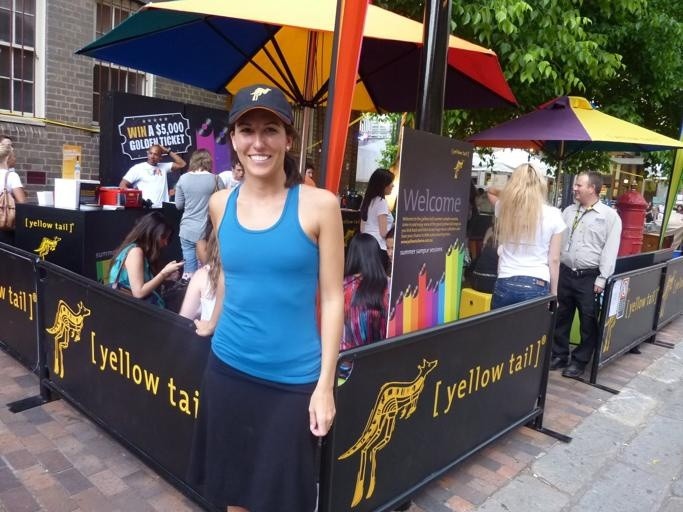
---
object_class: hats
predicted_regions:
[228,84,292,125]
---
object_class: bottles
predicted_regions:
[74,155,80,179]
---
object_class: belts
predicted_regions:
[563,265,599,278]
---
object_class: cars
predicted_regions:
[672,192,682,211]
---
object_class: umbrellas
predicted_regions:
[462,96,683,208]
[73,1,523,184]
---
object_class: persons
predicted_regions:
[0,134,27,245]
[174,148,243,323]
[117,142,186,212]
[108,209,188,311]
[337,233,392,382]
[382,227,396,265]
[192,81,344,511]
[462,160,624,381]
[305,164,315,179]
[358,167,395,271]
[643,201,683,226]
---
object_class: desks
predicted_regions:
[17,203,163,278]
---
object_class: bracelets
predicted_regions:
[167,149,172,156]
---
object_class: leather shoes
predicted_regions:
[563,361,585,378]
[550,357,568,369]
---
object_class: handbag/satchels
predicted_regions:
[112,284,133,297]
[0,190,16,229]
